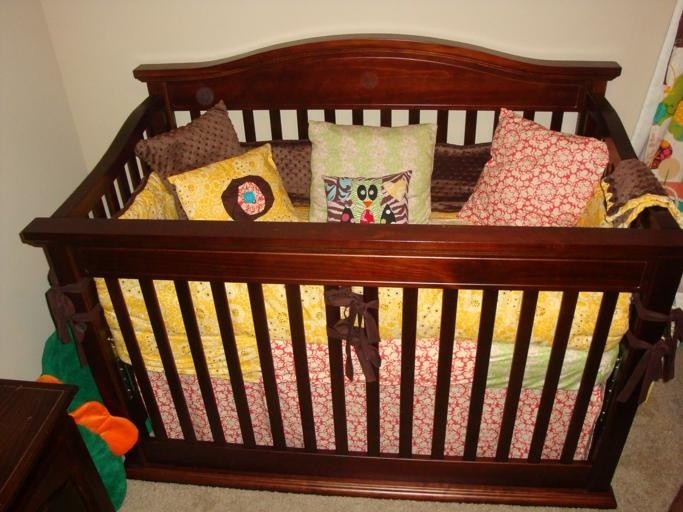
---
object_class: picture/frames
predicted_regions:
[627,1,683,309]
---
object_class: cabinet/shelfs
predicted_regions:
[0,377,114,512]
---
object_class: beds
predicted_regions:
[17,28,682,512]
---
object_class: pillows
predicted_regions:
[165,142,298,221]
[301,118,437,224]
[135,99,240,175]
[453,102,609,227]
